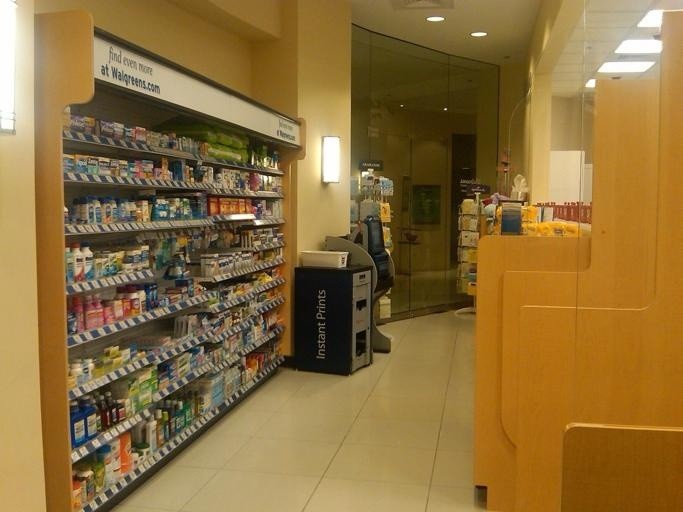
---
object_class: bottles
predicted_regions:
[186,165,190,180]
[189,167,194,183]
[73,432,132,511]
[66,283,158,334]
[64,195,142,223]
[65,242,94,283]
[130,391,203,457]
[231,363,254,389]
[70,390,127,448]
[152,198,170,219]
[535,200,591,224]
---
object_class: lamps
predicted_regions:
[321,135,340,184]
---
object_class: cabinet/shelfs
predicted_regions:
[39,10,306,512]
[475,11,681,512]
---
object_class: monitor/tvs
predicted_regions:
[361,215,385,253]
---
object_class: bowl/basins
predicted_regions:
[299,250,349,268]
